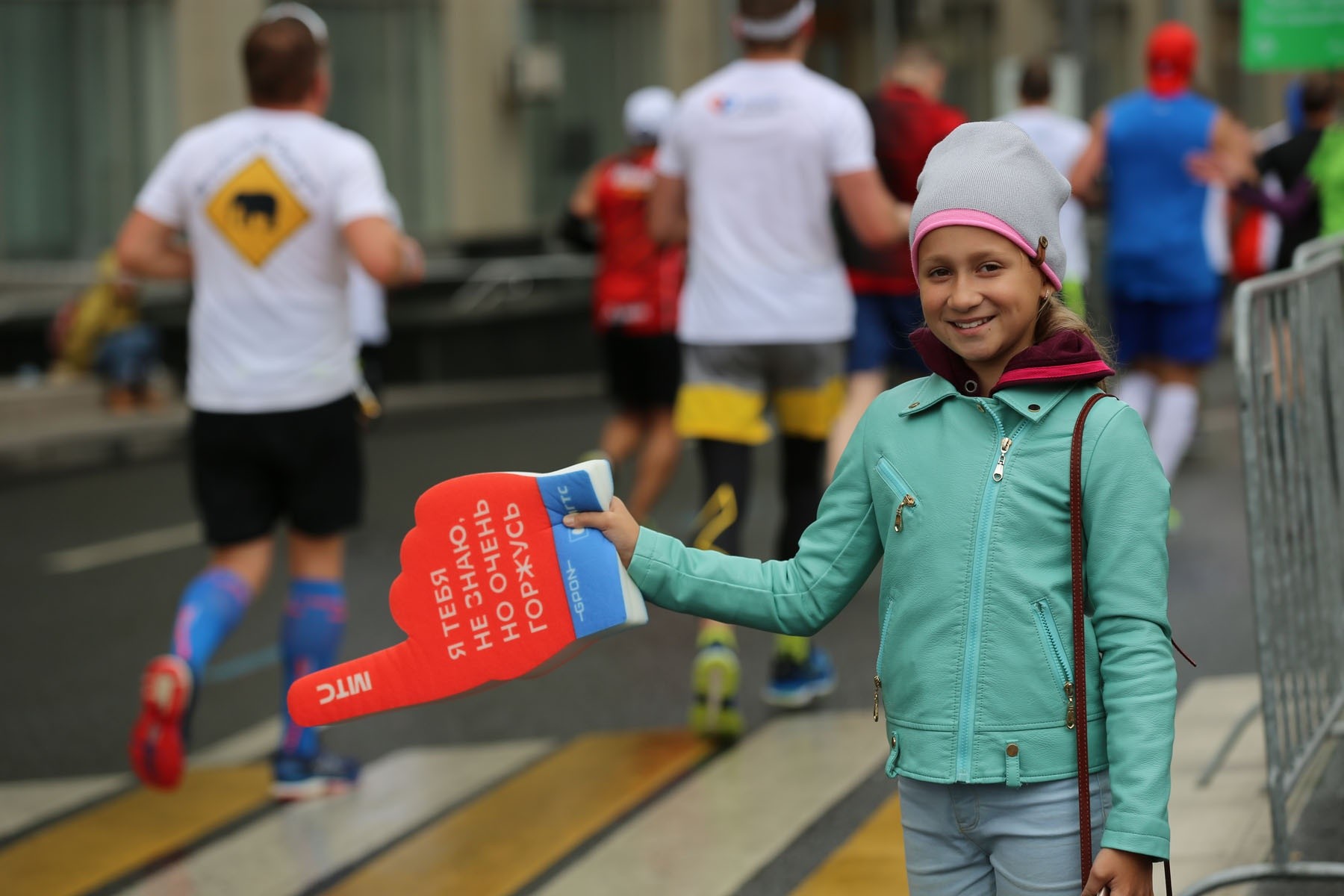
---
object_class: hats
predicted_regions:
[621,86,674,143]
[1144,25,1193,97]
[906,121,1073,289]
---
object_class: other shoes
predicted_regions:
[763,643,835,706]
[126,654,195,790]
[687,638,745,741]
[272,751,358,799]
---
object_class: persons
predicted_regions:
[115,3,426,804]
[648,0,913,736]
[573,0,1344,736]
[1072,20,1260,485]
[562,121,1177,896]
